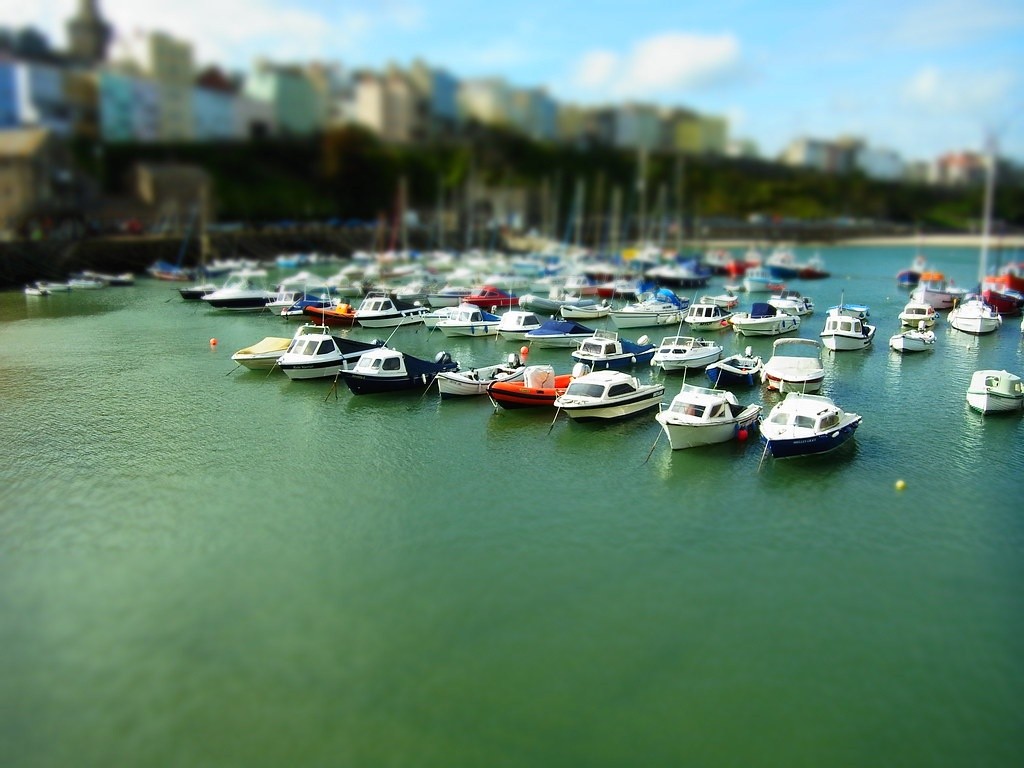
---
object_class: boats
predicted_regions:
[650,337,723,374]
[23,144,830,301]
[982,263,1024,315]
[768,289,814,316]
[233,336,294,369]
[462,288,520,308]
[436,301,501,338]
[898,300,939,328]
[607,288,689,330]
[820,316,876,351]
[266,288,332,317]
[758,392,864,459]
[517,287,595,314]
[656,383,764,450]
[895,257,928,287]
[888,321,937,353]
[559,300,611,320]
[496,311,543,341]
[554,369,666,422]
[947,295,1003,334]
[524,317,593,349]
[436,353,526,399]
[765,338,825,393]
[705,346,765,386]
[909,273,968,309]
[487,362,592,410]
[303,303,358,325]
[684,304,733,331]
[570,328,656,369]
[728,301,801,337]
[428,285,471,306]
[701,291,738,308]
[202,269,301,310]
[825,290,869,320]
[421,307,457,330]
[967,369,1024,416]
[338,347,460,395]
[353,291,431,328]
[279,325,388,381]
